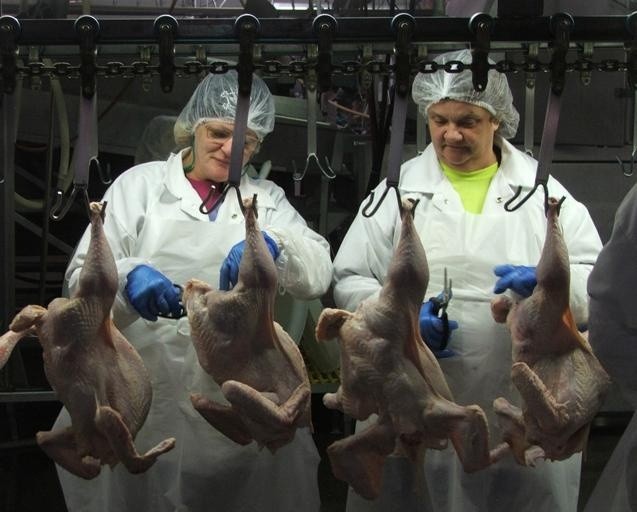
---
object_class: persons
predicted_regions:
[586,179,637,512]
[47,68,335,512]
[330,47,602,512]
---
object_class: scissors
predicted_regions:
[154,281,188,318]
[428,267,452,353]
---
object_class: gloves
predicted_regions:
[219,229,280,291]
[417,287,460,359]
[123,264,188,322]
[493,262,540,300]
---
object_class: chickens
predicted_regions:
[314,198,511,502]
[491,197,612,469]
[3,201,177,481]
[181,198,311,450]
